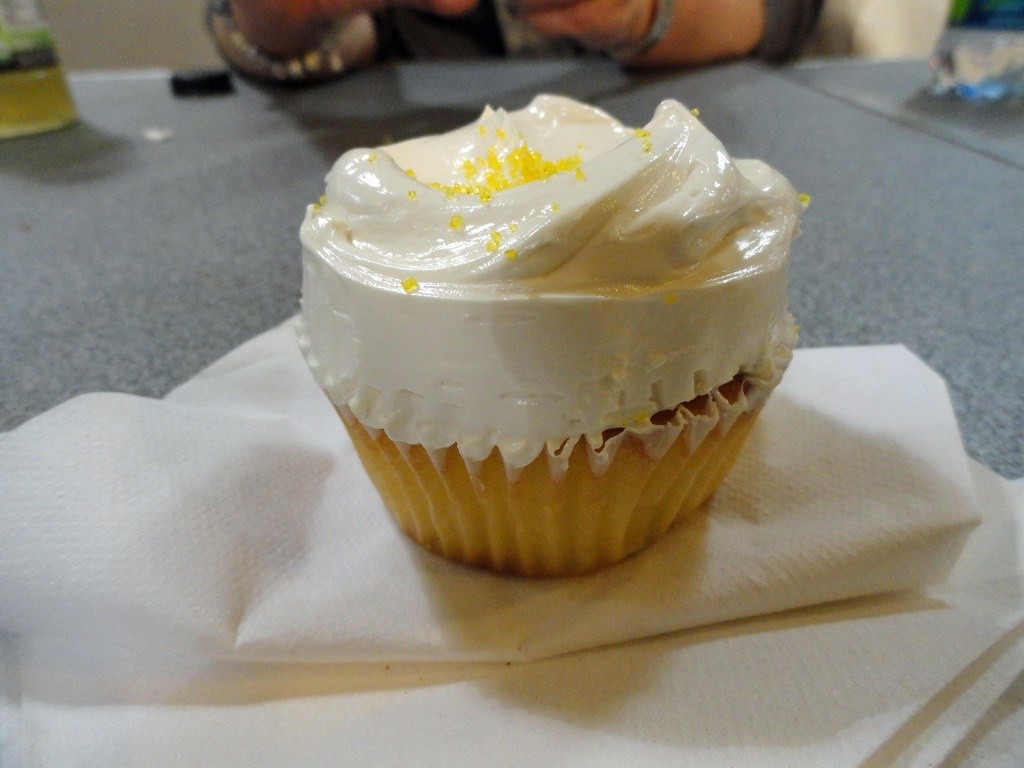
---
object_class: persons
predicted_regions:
[206,0,827,84]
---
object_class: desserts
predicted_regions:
[289,91,802,578]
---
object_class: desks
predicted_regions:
[1,66,1024,768]
[787,58,1024,171]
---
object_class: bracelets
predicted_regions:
[213,0,339,76]
[608,0,672,61]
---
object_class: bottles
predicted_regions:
[930,0,1024,107]
[0,0,78,140]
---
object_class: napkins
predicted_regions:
[0,315,1024,768]
